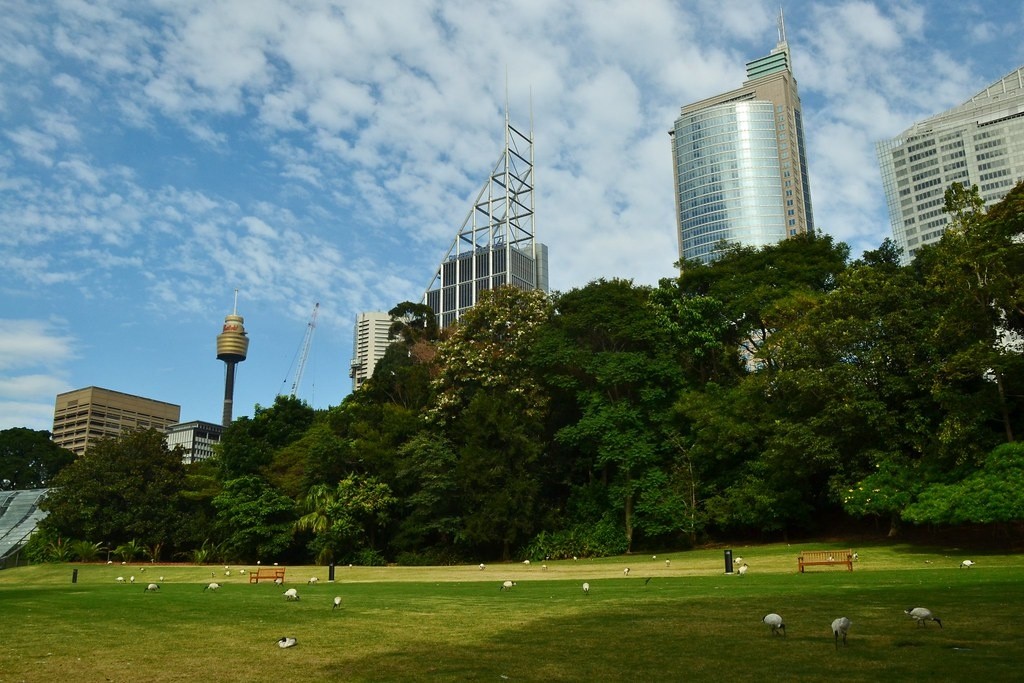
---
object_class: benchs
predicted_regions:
[798,548,852,573]
[250,568,286,583]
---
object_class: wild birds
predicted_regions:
[761,612,787,641]
[273,636,297,648]
[831,617,850,652]
[904,606,943,631]
[331,596,342,612]
[106,559,353,600]
[734,555,743,566]
[736,563,748,576]
[960,559,975,570]
[827,551,859,562]
[478,553,671,597]
